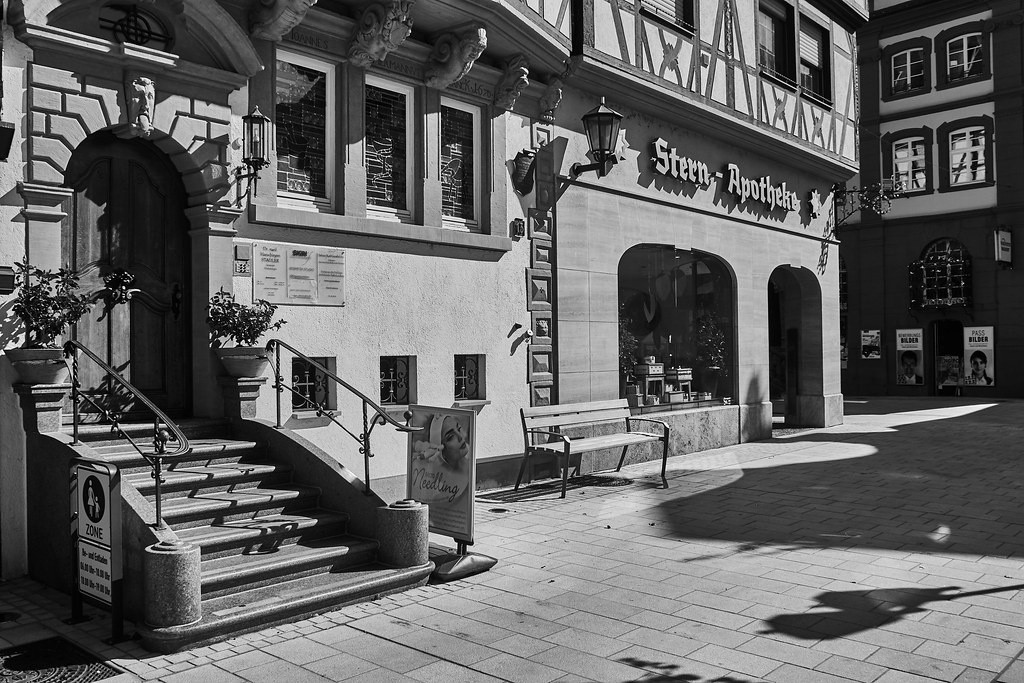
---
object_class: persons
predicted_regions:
[898,350,923,385]
[424,414,469,513]
[965,350,993,386]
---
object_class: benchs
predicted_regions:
[514,397,670,497]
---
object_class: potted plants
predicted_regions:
[205,286,288,377]
[697,317,728,398]
[2,256,98,384]
[618,301,640,399]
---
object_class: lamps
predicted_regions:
[573,102,624,177]
[236,104,272,210]
